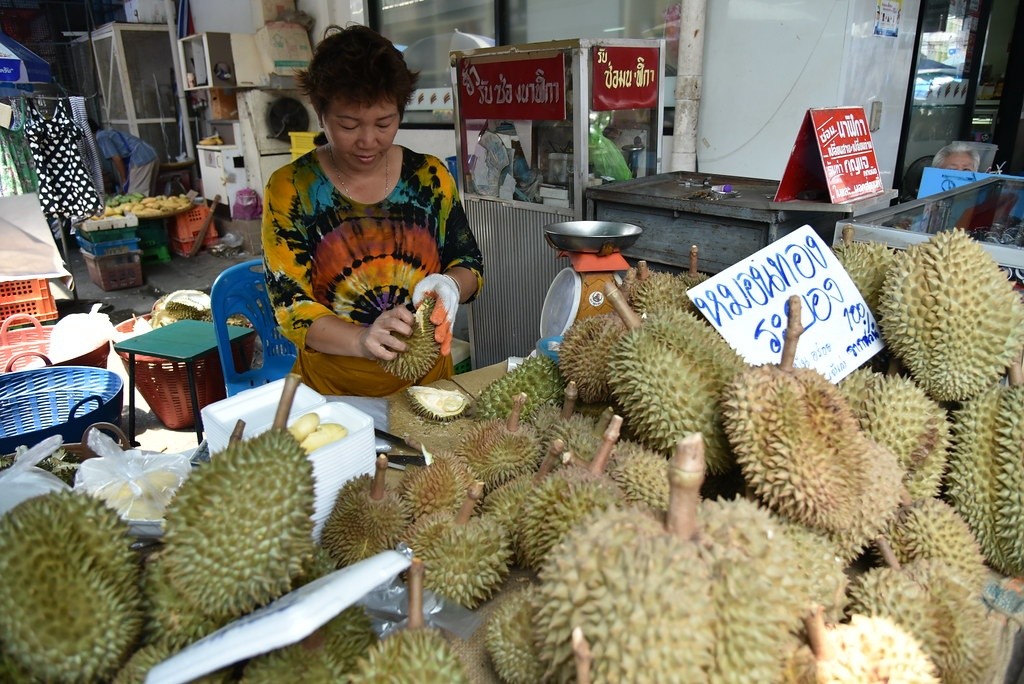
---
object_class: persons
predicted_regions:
[86,117,160,199]
[934,143,981,174]
[262,25,486,404]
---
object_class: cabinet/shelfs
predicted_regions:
[71,33,107,128]
[196,144,247,219]
[91,22,196,176]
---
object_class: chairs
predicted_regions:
[210,258,299,399]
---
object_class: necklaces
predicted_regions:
[329,145,388,200]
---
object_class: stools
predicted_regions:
[113,320,255,450]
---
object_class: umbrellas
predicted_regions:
[0,32,53,103]
[400,28,495,75]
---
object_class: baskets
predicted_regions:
[113,313,258,428]
[0,366,123,456]
[0,313,110,374]
[0,423,144,458]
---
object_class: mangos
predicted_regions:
[92,192,191,220]
[198,139,224,146]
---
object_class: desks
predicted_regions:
[156,159,195,189]
[0,359,518,684]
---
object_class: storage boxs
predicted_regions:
[200,375,379,549]
[208,89,238,120]
[253,21,316,90]
[177,31,269,91]
[75,205,219,293]
[0,277,59,329]
[251,0,295,29]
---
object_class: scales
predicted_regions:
[535,218,643,340]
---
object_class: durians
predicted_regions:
[0,227,1024,684]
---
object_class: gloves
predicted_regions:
[412,272,460,355]
